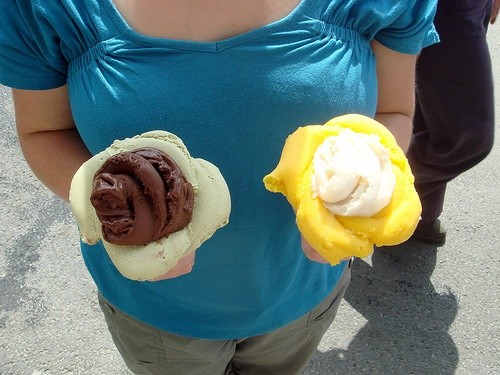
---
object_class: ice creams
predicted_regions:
[263,114,422,265]
[67,129,231,281]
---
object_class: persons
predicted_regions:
[0,0,440,374]
[403,0,500,246]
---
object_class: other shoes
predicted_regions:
[416,218,446,245]
